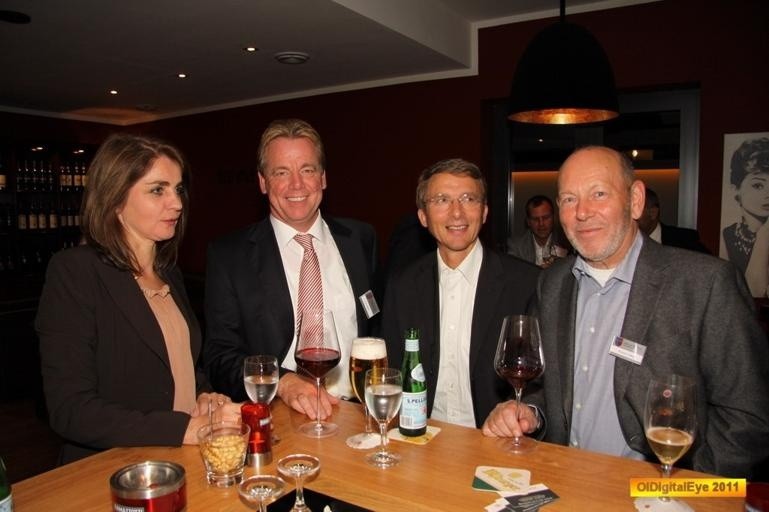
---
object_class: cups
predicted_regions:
[199,417,249,484]
[241,404,272,465]
[541,248,557,267]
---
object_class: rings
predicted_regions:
[217,402,225,405]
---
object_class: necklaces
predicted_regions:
[735,223,757,256]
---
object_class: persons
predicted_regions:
[368,158,543,431]
[638,188,709,254]
[199,117,380,421]
[504,195,571,270]
[719,138,769,298]
[32,134,275,465]
[481,145,769,478]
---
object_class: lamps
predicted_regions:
[502,0,623,127]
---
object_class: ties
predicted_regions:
[293,234,326,392]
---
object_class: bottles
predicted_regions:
[398,328,428,435]
[110,459,189,511]
[0,158,88,275]
[0,450,17,511]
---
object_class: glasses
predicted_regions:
[423,193,482,205]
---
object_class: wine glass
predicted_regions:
[244,353,284,443]
[293,309,342,438]
[240,452,323,511]
[494,314,544,455]
[365,367,403,467]
[347,337,391,451]
[633,372,696,511]
